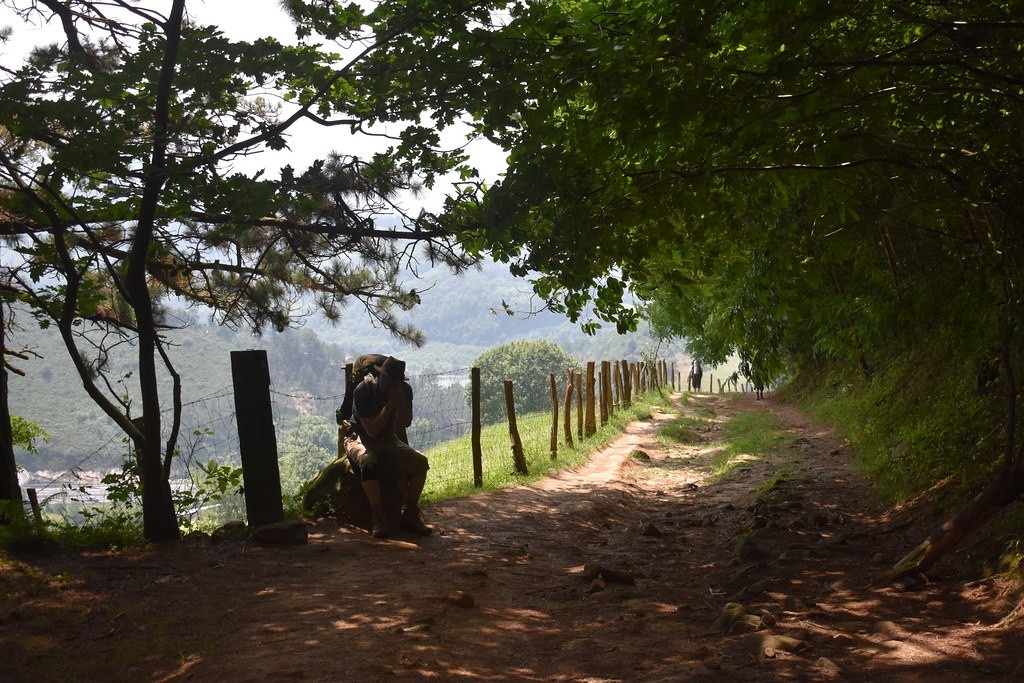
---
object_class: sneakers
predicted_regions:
[403,507,433,535]
[372,509,388,540]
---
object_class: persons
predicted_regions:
[342,356,436,539]
[688,359,702,394]
[754,359,767,400]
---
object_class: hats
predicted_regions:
[374,355,410,382]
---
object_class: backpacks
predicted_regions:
[336,353,408,427]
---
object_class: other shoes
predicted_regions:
[760,396,763,399]
[756,397,759,400]
